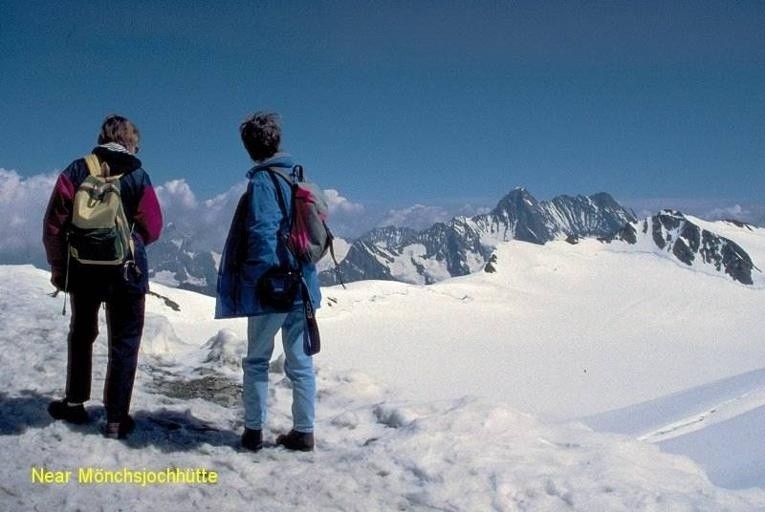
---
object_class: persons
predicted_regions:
[216,107,322,452]
[42,115,162,442]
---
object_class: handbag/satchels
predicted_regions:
[254,266,300,313]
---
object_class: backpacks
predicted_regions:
[68,153,131,265]
[264,164,334,264]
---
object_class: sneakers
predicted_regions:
[277,428,314,452]
[241,426,263,450]
[47,398,90,425]
[106,413,135,440]
